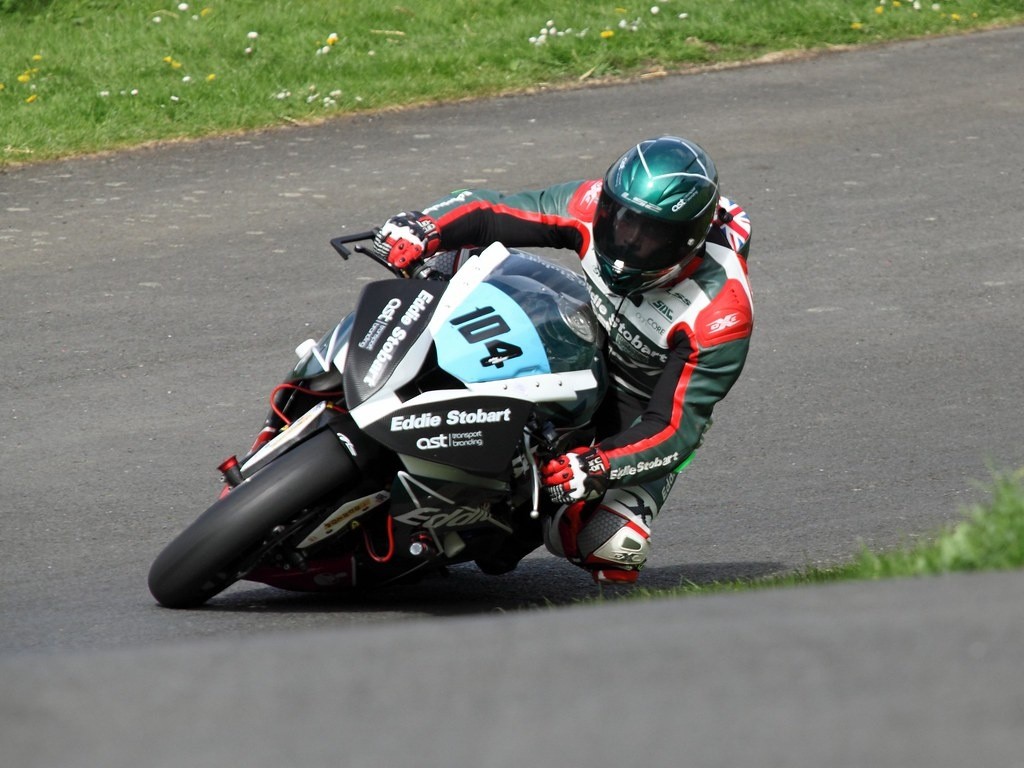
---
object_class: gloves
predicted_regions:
[373,210,440,269]
[540,445,612,503]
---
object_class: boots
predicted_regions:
[475,492,569,576]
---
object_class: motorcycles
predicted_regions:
[145,192,752,610]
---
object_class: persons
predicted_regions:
[375,137,754,585]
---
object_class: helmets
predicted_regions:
[591,141,720,296]
